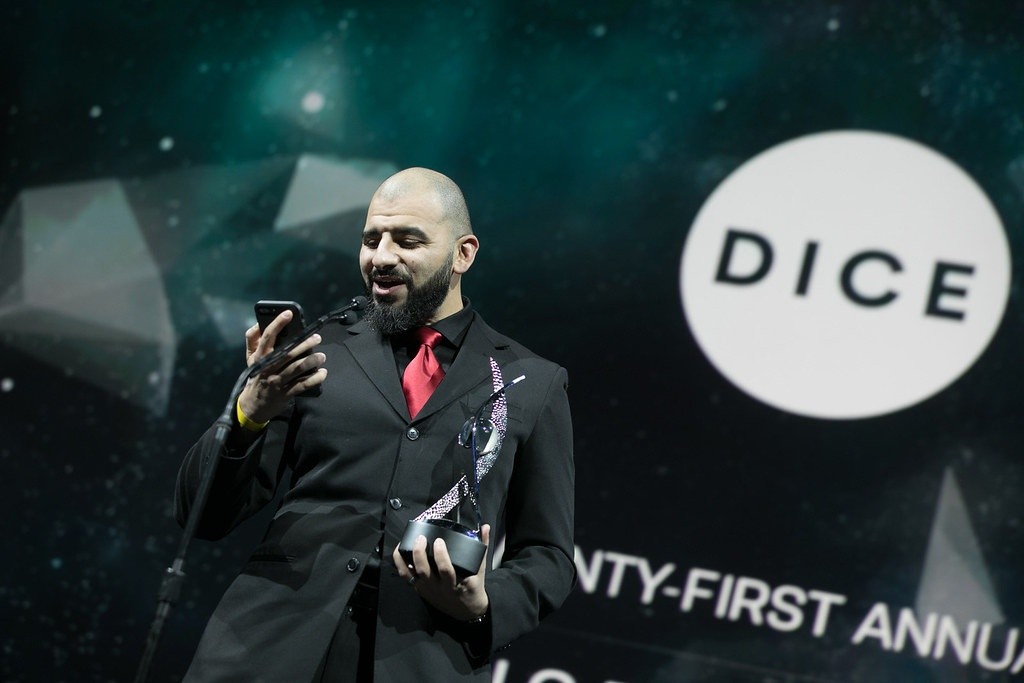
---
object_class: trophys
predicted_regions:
[398,356,525,576]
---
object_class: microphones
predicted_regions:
[217,296,368,427]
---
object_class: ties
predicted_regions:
[401,326,444,421]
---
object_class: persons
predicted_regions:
[173,167,575,683]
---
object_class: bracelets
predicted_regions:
[470,614,487,626]
[237,400,269,430]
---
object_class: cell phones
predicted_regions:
[254,299,322,398]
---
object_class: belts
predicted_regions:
[348,582,380,612]
